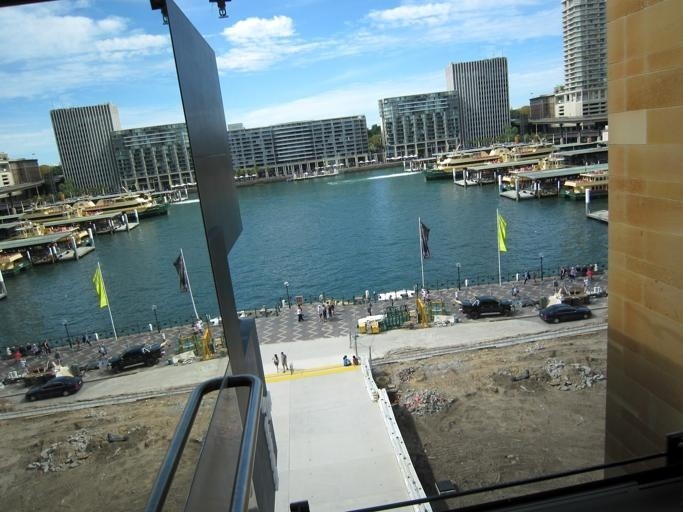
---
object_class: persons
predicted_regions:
[5,339,51,367]
[192,316,204,336]
[420,288,431,307]
[353,356,358,365]
[523,270,530,285]
[297,304,304,323]
[54,348,61,366]
[18,358,29,378]
[148,321,153,333]
[511,283,519,297]
[552,262,598,293]
[317,301,336,322]
[261,299,282,318]
[281,352,288,373]
[366,298,373,316]
[343,356,351,366]
[272,354,279,372]
[76,331,109,359]
[160,331,166,343]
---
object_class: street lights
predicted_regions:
[283,282,292,310]
[61,320,73,350]
[413,283,421,325]
[456,262,462,290]
[539,253,545,281]
[151,304,161,333]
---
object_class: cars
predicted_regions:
[24,374,84,401]
[108,343,166,372]
[538,304,591,325]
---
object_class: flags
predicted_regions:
[497,211,507,252]
[92,264,109,309]
[173,252,190,291]
[419,221,431,259]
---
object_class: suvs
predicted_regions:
[460,295,512,319]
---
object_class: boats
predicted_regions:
[418,141,607,200]
[0,185,169,276]
